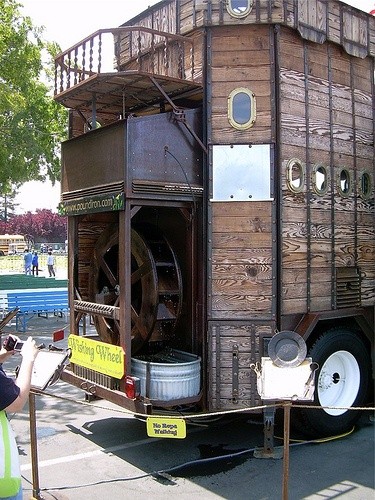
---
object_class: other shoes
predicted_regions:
[49,276,51,277]
[54,274,55,276]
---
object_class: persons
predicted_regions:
[0,334,41,500]
[46,251,55,277]
[23,249,33,275]
[31,252,39,275]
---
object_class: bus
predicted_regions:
[0,234,29,256]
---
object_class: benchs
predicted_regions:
[0,290,69,332]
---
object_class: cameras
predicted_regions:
[13,341,26,351]
